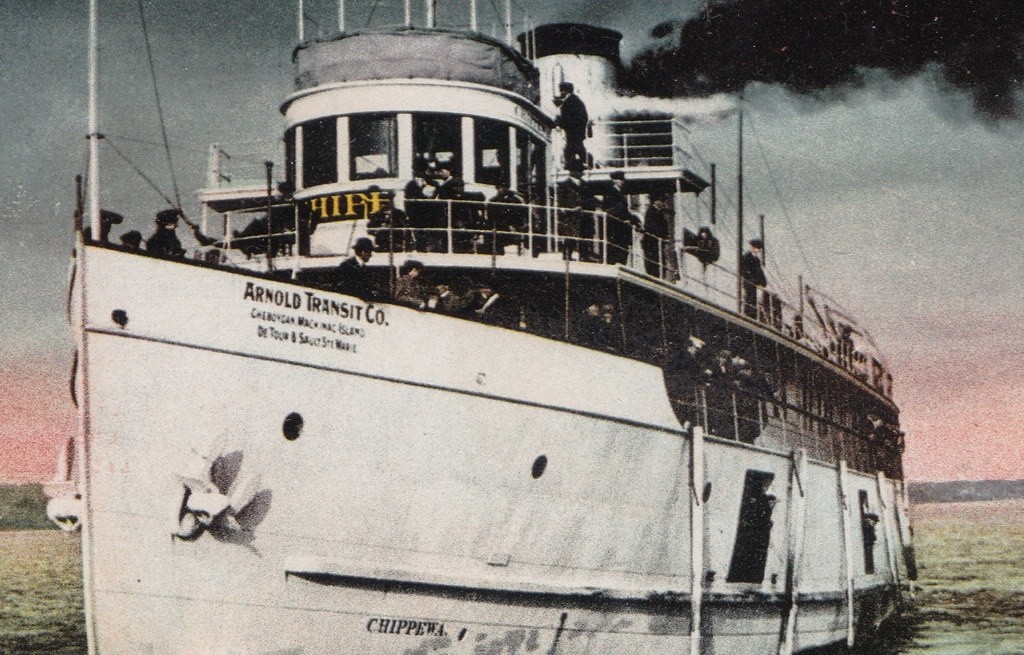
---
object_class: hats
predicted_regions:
[716,347,732,357]
[493,178,510,188]
[121,231,143,245]
[379,191,395,202]
[411,158,427,170]
[354,239,374,249]
[156,209,181,225]
[650,192,666,202]
[751,239,765,248]
[687,336,706,349]
[478,275,499,291]
[564,160,587,170]
[611,172,624,180]
[437,163,454,171]
[582,298,601,307]
[277,180,296,193]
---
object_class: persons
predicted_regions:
[83,76,903,478]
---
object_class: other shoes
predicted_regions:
[743,312,758,319]
[564,253,572,260]
[579,257,595,263]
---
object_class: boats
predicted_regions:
[41,0,914,654]
